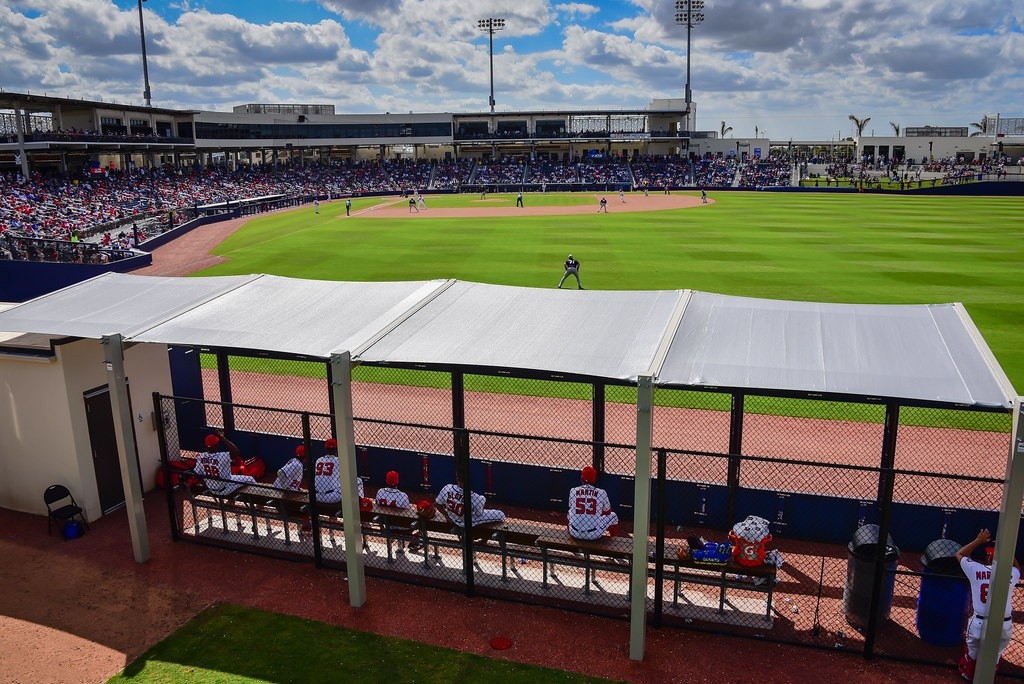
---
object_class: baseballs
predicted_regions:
[791,604,798,613]
[785,597,790,603]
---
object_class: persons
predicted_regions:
[9,126,163,143]
[596,197,608,214]
[628,151,794,203]
[373,470,427,550]
[312,436,366,506]
[566,466,620,541]
[433,152,524,199]
[0,170,190,263]
[270,445,312,533]
[434,469,506,544]
[797,151,1024,190]
[516,193,523,207]
[302,158,432,216]
[472,128,677,138]
[139,157,302,216]
[619,189,625,203]
[193,427,259,498]
[954,529,1021,683]
[527,153,631,192]
[557,254,584,289]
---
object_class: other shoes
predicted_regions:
[959,667,971,681]
[409,542,425,550]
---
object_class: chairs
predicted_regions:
[44,484,91,540]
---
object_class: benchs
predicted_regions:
[181,470,778,633]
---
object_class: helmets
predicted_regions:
[985,540,996,565]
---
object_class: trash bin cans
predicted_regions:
[843,525,901,628]
[914,539,973,645]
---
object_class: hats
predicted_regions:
[296,446,304,457]
[582,466,597,483]
[325,439,337,448]
[205,435,220,448]
[417,500,431,511]
[385,471,399,486]
[360,498,373,513]
